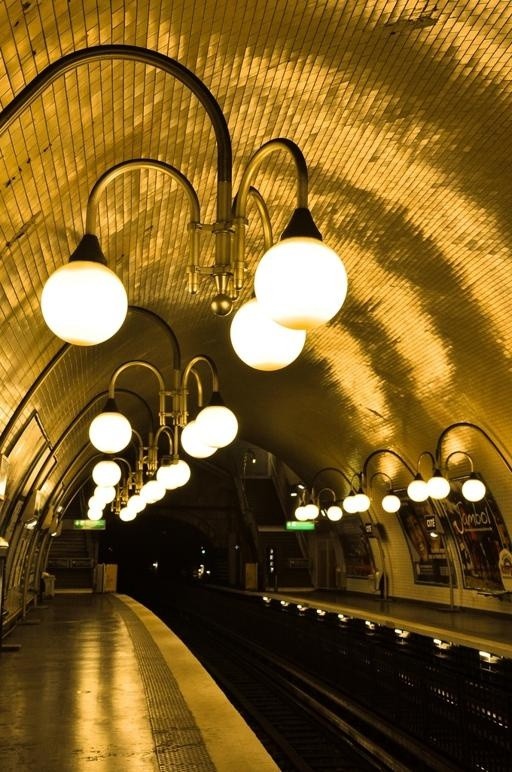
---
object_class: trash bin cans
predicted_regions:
[40,576,55,599]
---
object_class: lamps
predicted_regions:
[351,420,510,517]
[0,307,238,460]
[0,39,348,374]
[0,388,190,628]
